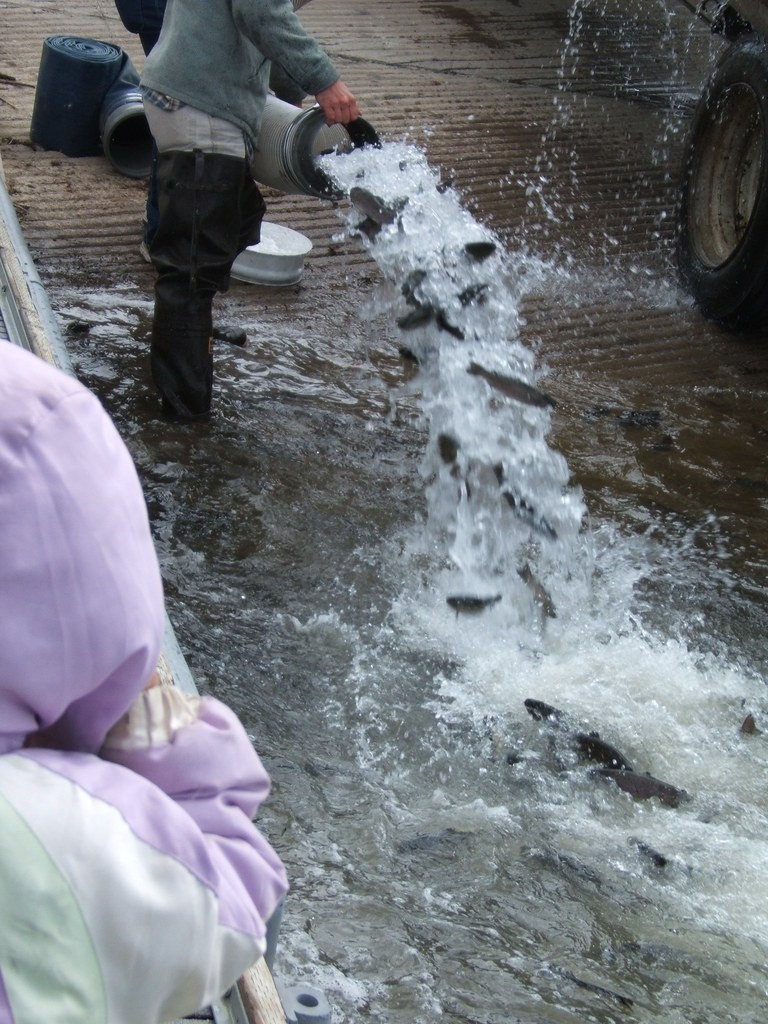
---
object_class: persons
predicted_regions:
[0,338,288,1024]
[114,0,359,424]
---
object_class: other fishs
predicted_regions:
[322,153,714,892]
